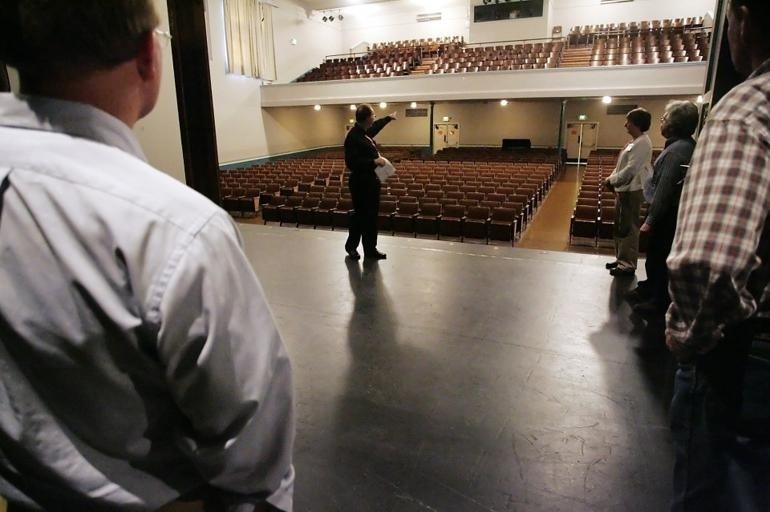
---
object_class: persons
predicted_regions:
[623,101,699,316]
[603,91,654,277]
[1,1,296,511]
[665,0,770,511]
[345,104,398,259]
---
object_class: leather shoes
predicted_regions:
[345,246,360,260]
[629,288,644,299]
[366,250,387,259]
[607,262,617,268]
[634,302,664,312]
[611,268,635,276]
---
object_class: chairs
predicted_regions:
[294,26,565,82]
[220,148,567,249]
[570,149,662,249]
[567,14,710,67]
